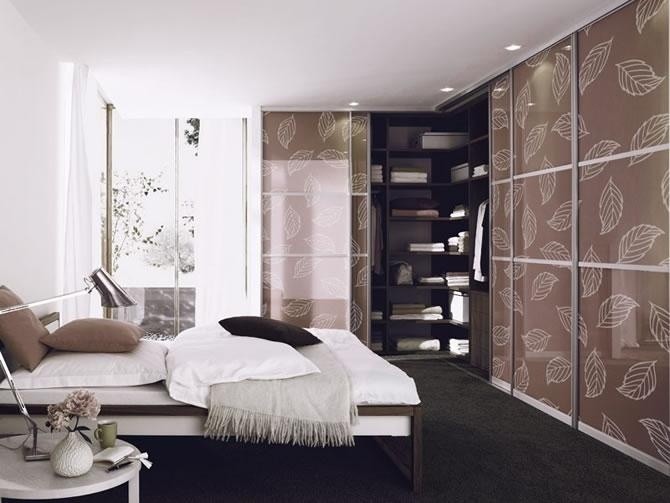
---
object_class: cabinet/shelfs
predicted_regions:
[490,0,670,475]
[371,109,469,356]
[467,88,491,379]
[257,106,373,351]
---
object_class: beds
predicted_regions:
[0,310,426,495]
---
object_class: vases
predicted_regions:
[49,428,95,479]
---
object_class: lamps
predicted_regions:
[82,266,140,310]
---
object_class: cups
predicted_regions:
[94,418,118,447]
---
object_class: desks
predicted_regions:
[0,428,145,501]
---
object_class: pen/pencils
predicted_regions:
[106,455,128,473]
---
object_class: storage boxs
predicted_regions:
[404,127,469,152]
[448,160,469,183]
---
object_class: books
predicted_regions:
[93,446,135,465]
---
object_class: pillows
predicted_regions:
[37,316,149,353]
[218,313,323,347]
[0,332,169,390]
[1,282,53,374]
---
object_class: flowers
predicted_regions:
[47,389,104,433]
